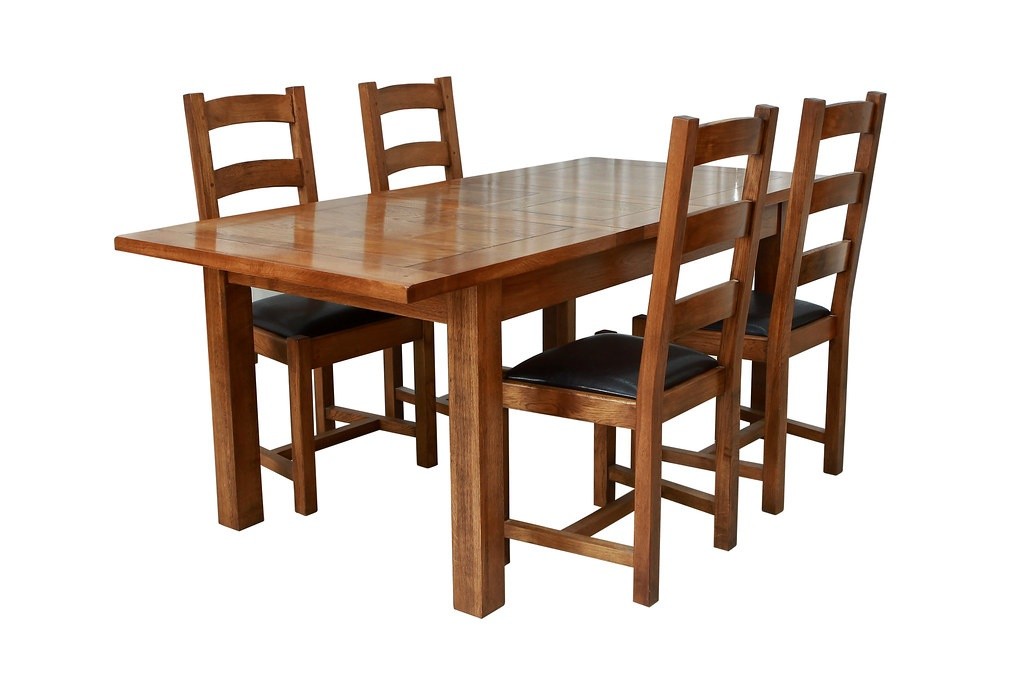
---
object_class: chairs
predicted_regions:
[503,104,780,610]
[358,77,558,421]
[632,92,886,511]
[183,86,437,515]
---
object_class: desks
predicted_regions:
[113,158,826,618]
